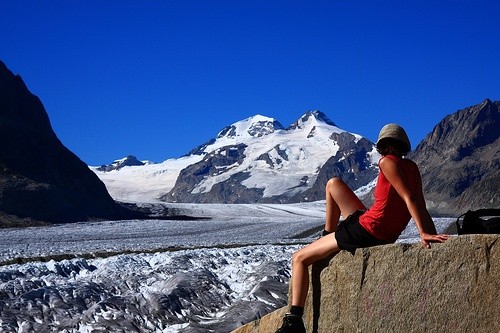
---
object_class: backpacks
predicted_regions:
[456,208,500,235]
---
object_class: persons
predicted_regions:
[273,123,450,333]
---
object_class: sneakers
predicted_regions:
[274,314,307,333]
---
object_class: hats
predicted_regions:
[376,124,412,152]
